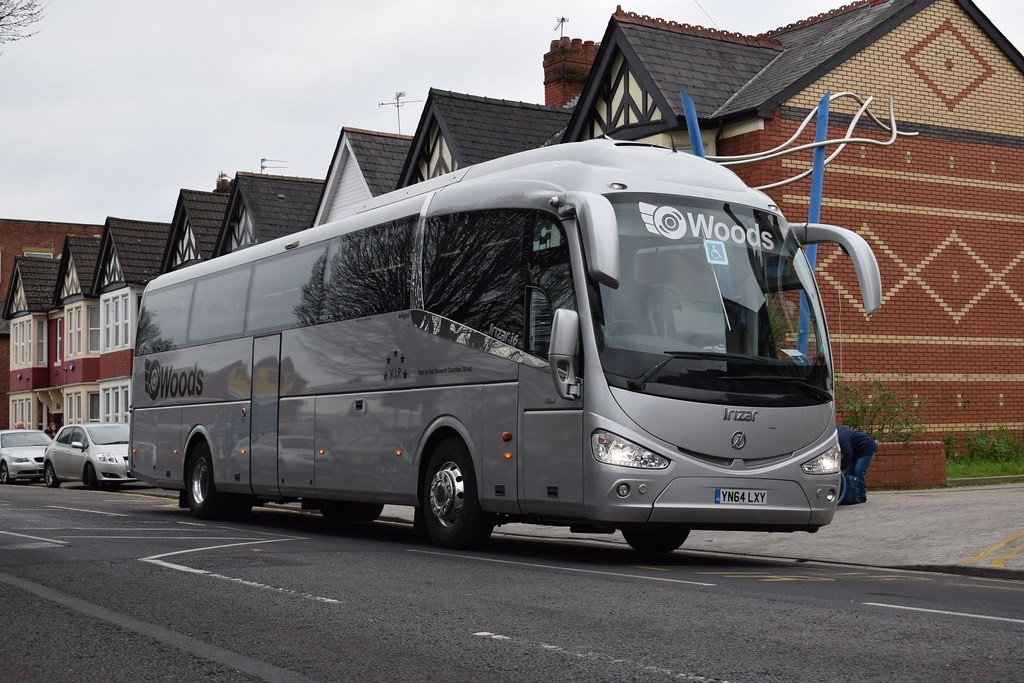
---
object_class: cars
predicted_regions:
[43,422,138,490]
[0,428,52,484]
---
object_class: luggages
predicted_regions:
[840,475,858,505]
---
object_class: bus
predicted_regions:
[128,138,883,555]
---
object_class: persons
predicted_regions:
[49,423,57,438]
[837,426,879,504]
[17,422,25,429]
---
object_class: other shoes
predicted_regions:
[856,495,867,503]
